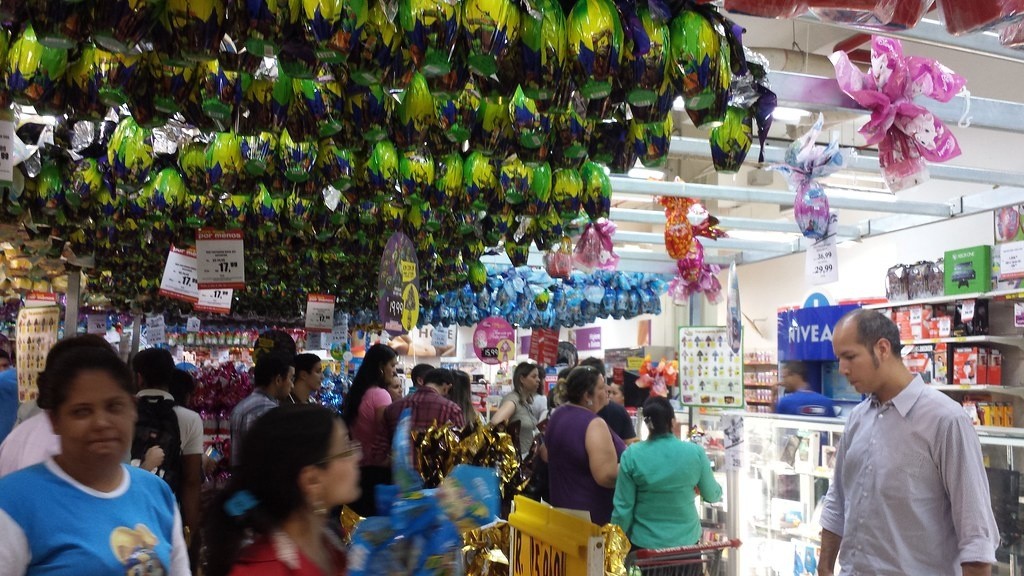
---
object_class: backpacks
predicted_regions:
[131,395,181,501]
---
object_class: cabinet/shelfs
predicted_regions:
[744,361,777,404]
[859,288,1024,439]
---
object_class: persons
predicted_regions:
[817,309,1000,576]
[0,329,722,576]
[774,361,836,417]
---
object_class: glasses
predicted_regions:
[314,440,362,464]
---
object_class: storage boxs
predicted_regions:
[740,244,1014,576]
